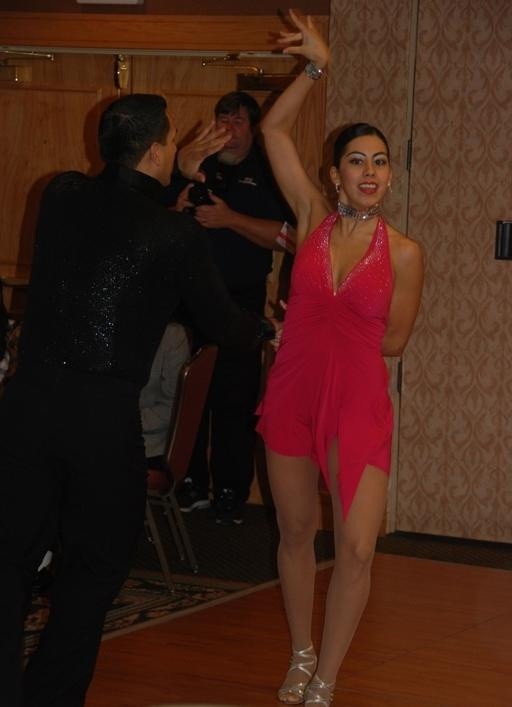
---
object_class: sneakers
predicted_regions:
[180,477,209,512]
[216,489,243,524]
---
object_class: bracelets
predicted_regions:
[304,60,324,81]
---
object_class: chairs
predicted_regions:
[141,344,218,595]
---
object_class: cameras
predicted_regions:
[188,183,208,206]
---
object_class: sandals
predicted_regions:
[277,642,317,704]
[304,673,336,707]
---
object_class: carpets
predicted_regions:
[23,576,233,656]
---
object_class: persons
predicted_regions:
[136,320,195,471]
[1,91,241,702]
[255,5,425,705]
[175,88,298,527]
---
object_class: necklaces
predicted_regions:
[335,198,384,222]
